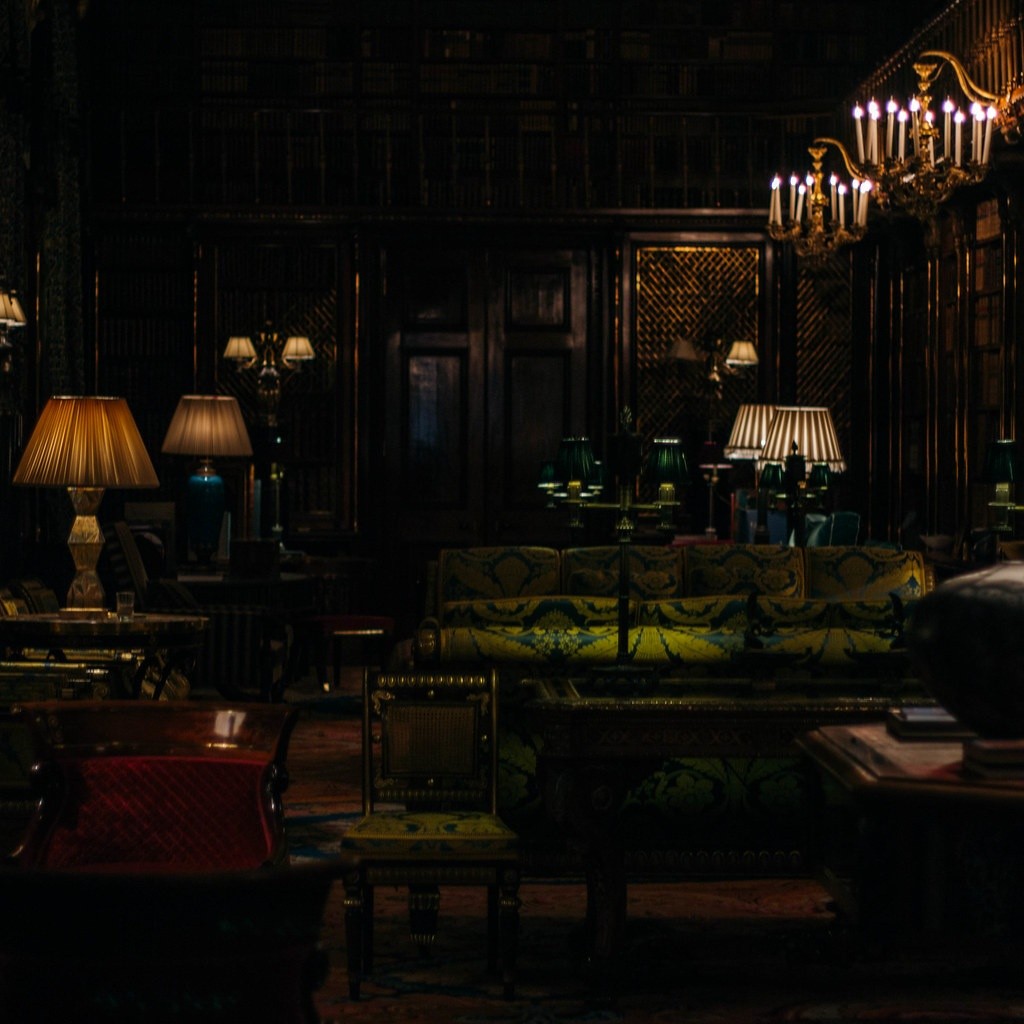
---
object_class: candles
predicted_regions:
[760,90,1000,272]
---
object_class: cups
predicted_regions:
[117,591,134,620]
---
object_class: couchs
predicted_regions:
[387,541,939,822]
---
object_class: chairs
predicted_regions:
[338,667,523,1002]
[0,648,336,1023]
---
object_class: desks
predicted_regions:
[796,720,1024,993]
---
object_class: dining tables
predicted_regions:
[0,611,212,638]
[526,675,946,1019]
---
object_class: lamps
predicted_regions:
[553,435,594,501]
[159,394,254,576]
[707,341,759,386]
[12,396,159,607]
[223,322,316,427]
[723,403,781,529]
[0,288,29,376]
[641,438,688,500]
[754,406,847,545]
